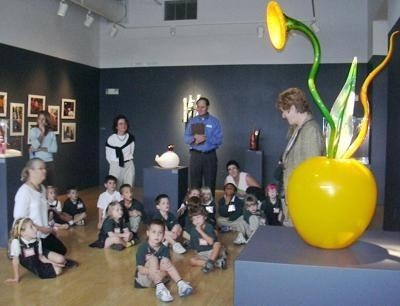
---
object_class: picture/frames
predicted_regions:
[0,91,8,118]
[48,105,60,134]
[27,95,46,118]
[27,121,38,145]
[61,98,77,120]
[9,102,24,137]
[61,122,77,144]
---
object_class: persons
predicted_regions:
[134,194,192,301]
[176,160,283,273]
[6,157,86,283]
[89,175,144,250]
[182,97,222,198]
[274,87,328,227]
[28,111,59,187]
[105,115,136,192]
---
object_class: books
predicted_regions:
[191,123,205,145]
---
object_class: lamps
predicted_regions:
[57,0,118,38]
[256,24,264,39]
[310,22,319,33]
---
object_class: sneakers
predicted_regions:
[173,242,186,253]
[233,233,246,244]
[111,243,124,251]
[178,281,192,297]
[129,237,139,246]
[76,220,85,226]
[156,287,173,302]
[215,259,226,269]
[64,260,77,267]
[221,225,231,233]
[201,261,214,273]
